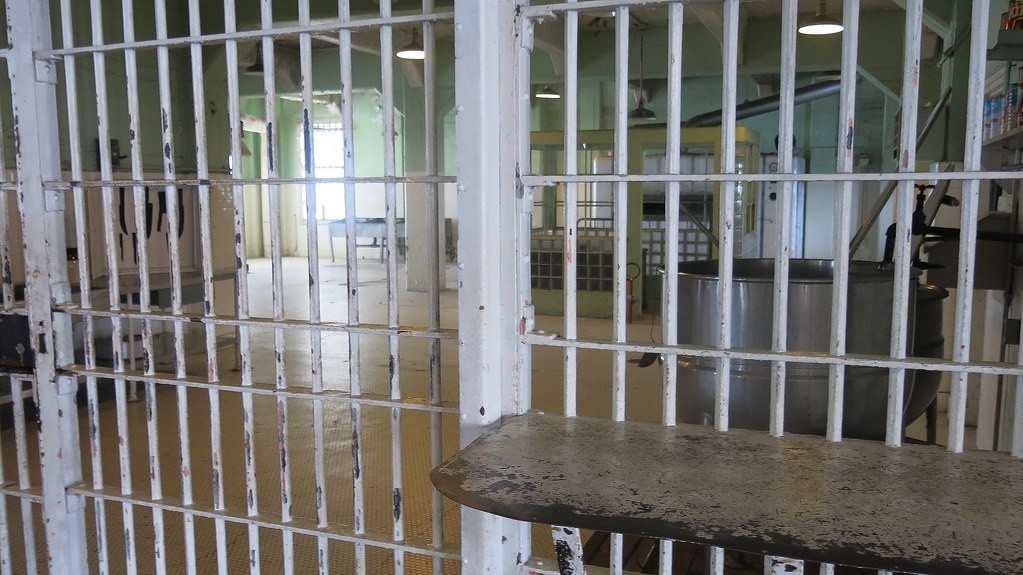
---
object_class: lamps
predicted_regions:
[243,44,265,75]
[627,31,657,120]
[796,1,846,36]
[533,50,562,99]
[396,25,426,60]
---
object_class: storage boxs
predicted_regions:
[898,284,951,429]
[657,256,922,436]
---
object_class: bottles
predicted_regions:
[990,95,1002,138]
[982,100,990,140]
[1006,83,1016,132]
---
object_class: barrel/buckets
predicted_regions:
[658,258,923,441]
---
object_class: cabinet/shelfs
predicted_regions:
[973,29,1023,156]
